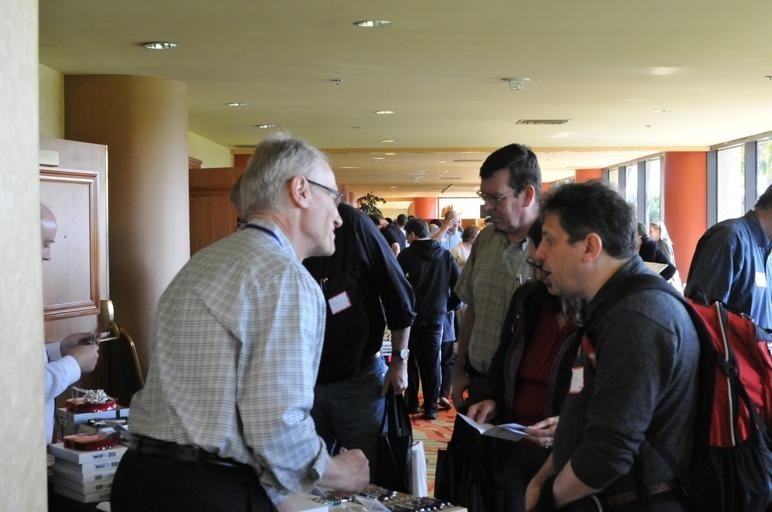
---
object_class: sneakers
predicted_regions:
[410,402,450,419]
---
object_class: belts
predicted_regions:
[129,437,242,467]
[604,482,676,507]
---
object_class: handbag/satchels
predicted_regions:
[375,435,429,498]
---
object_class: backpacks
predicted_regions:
[580,273,772,512]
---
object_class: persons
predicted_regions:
[231,143,771,512]
[109,129,370,512]
[40,199,100,456]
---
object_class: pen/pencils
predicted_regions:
[364,486,398,500]
[87,417,128,426]
[388,497,452,512]
[339,447,348,456]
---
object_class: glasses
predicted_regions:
[287,174,343,205]
[525,256,543,269]
[476,190,517,204]
